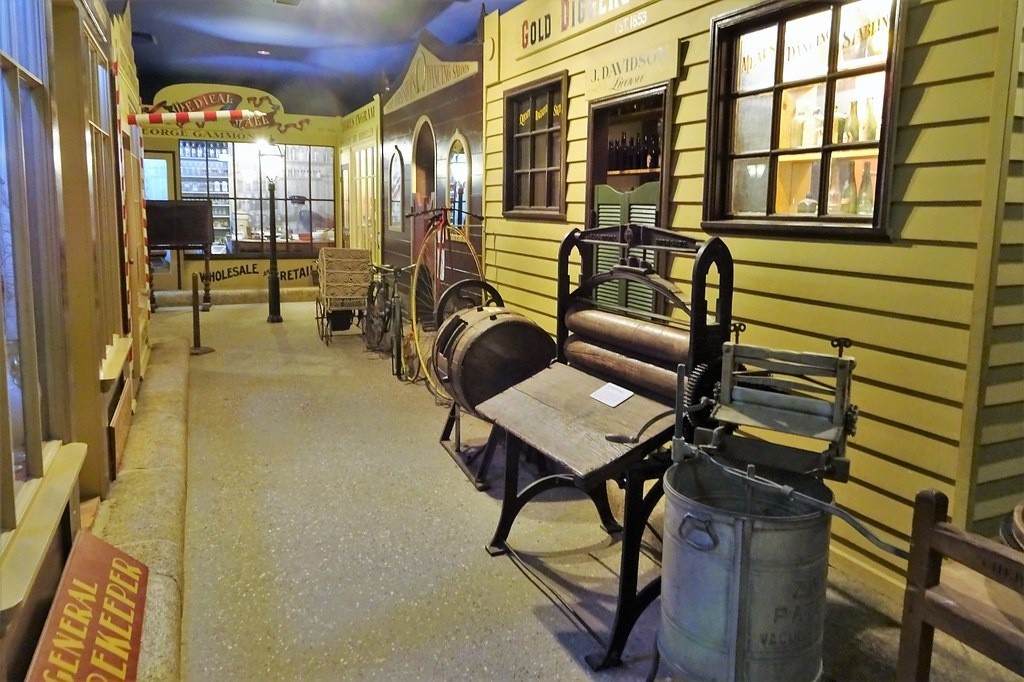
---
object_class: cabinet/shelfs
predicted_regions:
[181,142,231,253]
[605,97,662,177]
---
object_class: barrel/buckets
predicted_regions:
[650,453,848,682]
[430,301,557,419]
